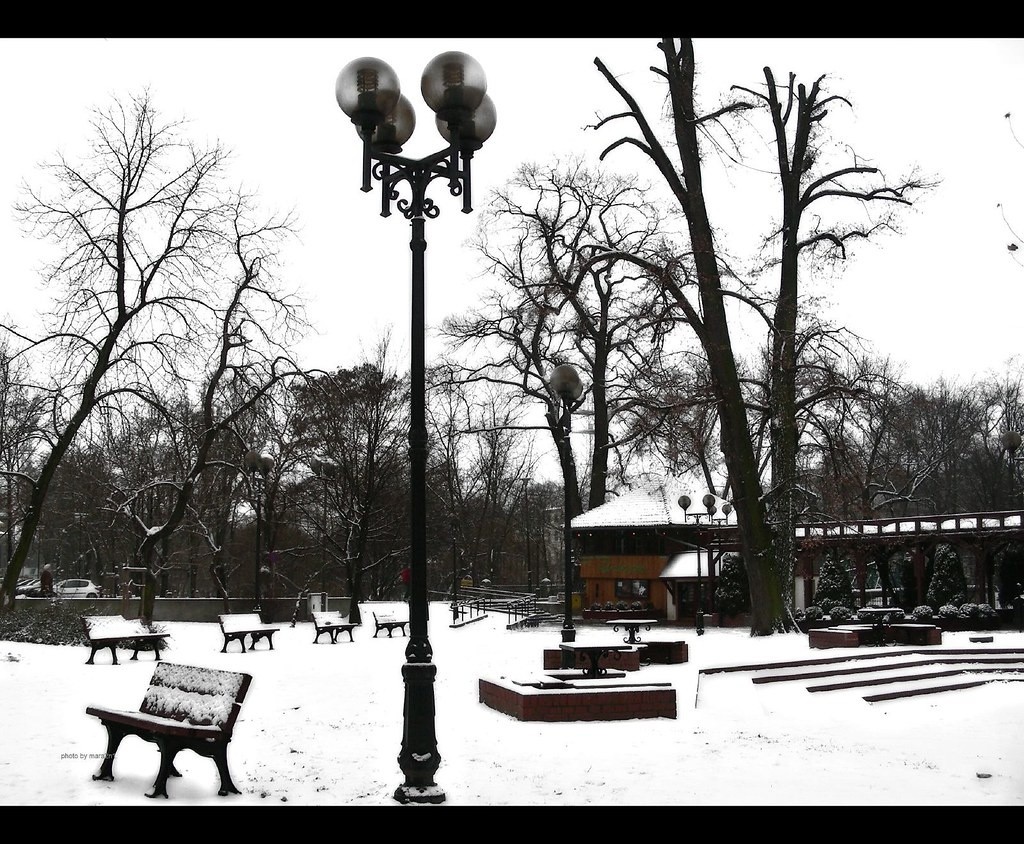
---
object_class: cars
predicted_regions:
[0,574,104,599]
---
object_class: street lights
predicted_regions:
[309,453,335,609]
[333,50,497,801]
[547,361,585,670]
[244,450,277,609]
[706,504,734,627]
[678,493,716,635]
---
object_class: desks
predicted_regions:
[559,642,633,679]
[606,619,658,667]
[858,608,903,647]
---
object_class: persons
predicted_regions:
[400,563,410,602]
[41,564,53,597]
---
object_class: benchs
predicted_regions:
[311,611,358,645]
[373,612,410,638]
[85,661,254,799]
[80,614,169,665]
[218,614,280,654]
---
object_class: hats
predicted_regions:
[44,564,50,571]
